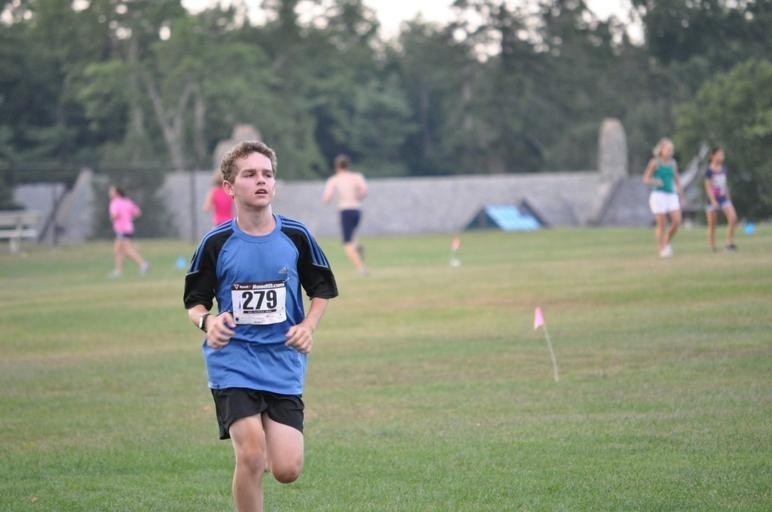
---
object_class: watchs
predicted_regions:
[199,311,211,334]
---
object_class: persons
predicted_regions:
[643,137,687,258]
[320,154,369,277]
[202,169,235,228]
[702,145,739,253]
[106,186,149,278]
[182,141,338,512]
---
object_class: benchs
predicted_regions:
[0,210,42,253]
